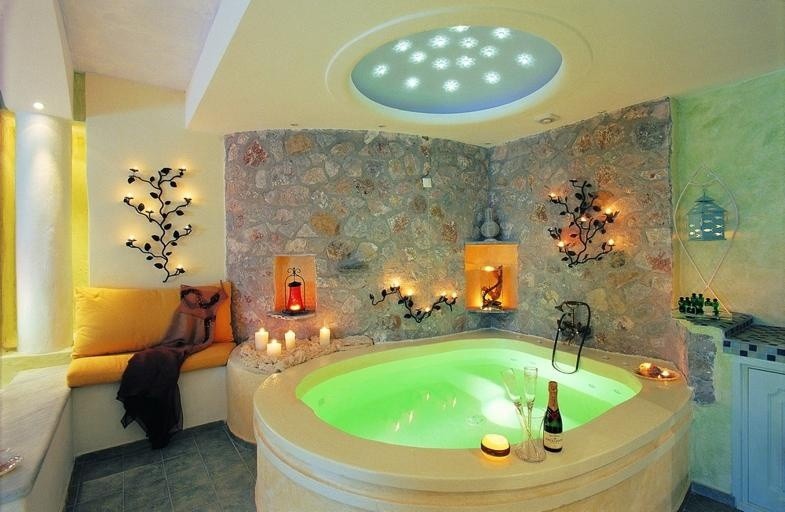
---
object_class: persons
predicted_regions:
[180,287,201,309]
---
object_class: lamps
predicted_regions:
[479,435,511,458]
[284,265,308,315]
[546,176,621,268]
[675,166,740,317]
[480,262,504,310]
[122,164,196,282]
[369,280,459,324]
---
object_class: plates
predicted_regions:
[632,367,680,381]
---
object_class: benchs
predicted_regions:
[0,362,75,511]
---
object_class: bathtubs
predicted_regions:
[250,325,692,511]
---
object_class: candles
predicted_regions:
[320,326,331,346]
[255,328,268,351]
[284,329,296,352]
[266,338,281,356]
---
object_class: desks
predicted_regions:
[226,337,374,445]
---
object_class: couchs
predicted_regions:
[66,281,238,459]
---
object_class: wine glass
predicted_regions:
[498,366,549,463]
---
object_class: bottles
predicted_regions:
[679,293,719,314]
[543,380,564,451]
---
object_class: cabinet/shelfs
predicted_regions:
[731,353,784,512]
